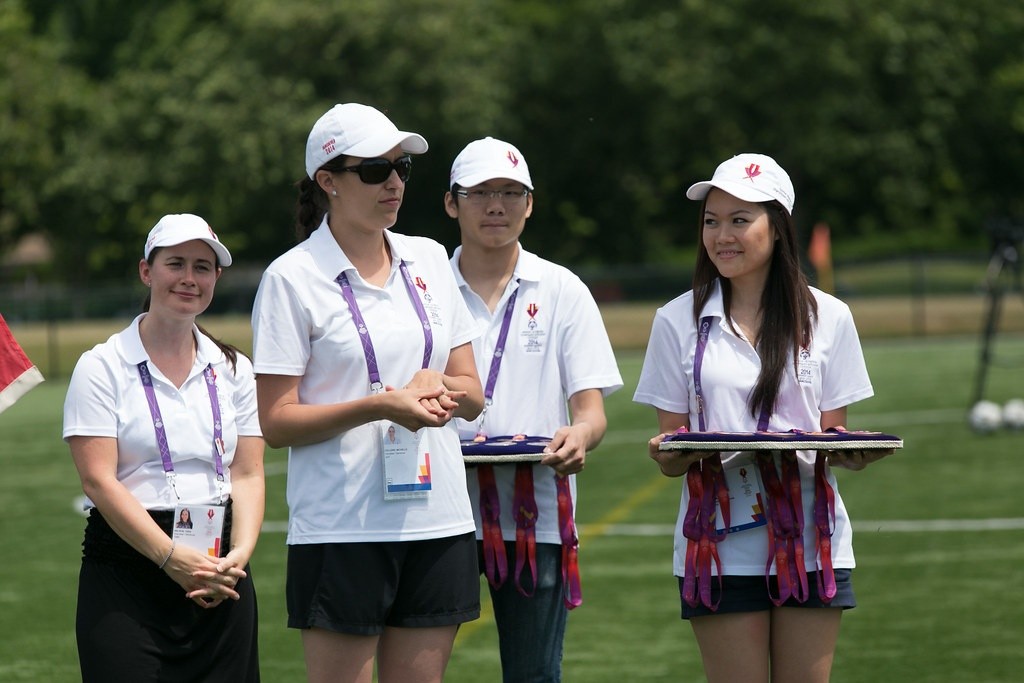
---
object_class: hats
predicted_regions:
[306,103,428,180]
[145,214,232,267]
[687,153,795,216]
[450,137,534,192]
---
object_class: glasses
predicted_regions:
[458,187,528,202]
[338,154,411,184]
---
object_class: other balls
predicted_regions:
[1002,400,1024,427]
[970,400,1002,431]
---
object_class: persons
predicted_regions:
[630,154,896,682]
[176,509,193,529]
[385,426,400,444]
[444,135,625,683]
[63,215,266,683]
[250,102,486,683]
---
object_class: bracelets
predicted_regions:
[158,542,176,572]
